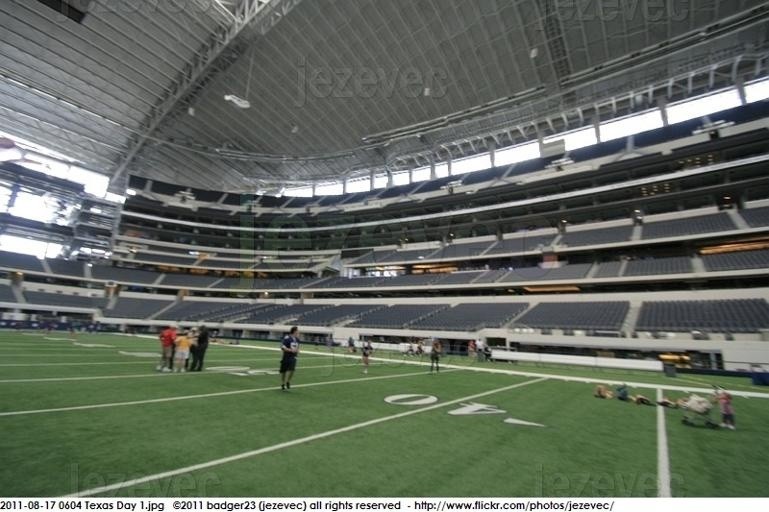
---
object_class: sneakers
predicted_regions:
[156,365,201,373]
[281,382,291,392]
[720,423,735,431]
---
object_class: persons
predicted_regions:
[415,345,425,357]
[467,340,478,362]
[189,325,208,371]
[429,336,442,375]
[714,387,736,430]
[404,346,414,357]
[280,327,301,394]
[186,326,199,369]
[360,339,374,374]
[474,337,488,364]
[155,323,179,373]
[173,329,192,373]
[594,382,712,414]
[348,336,356,353]
